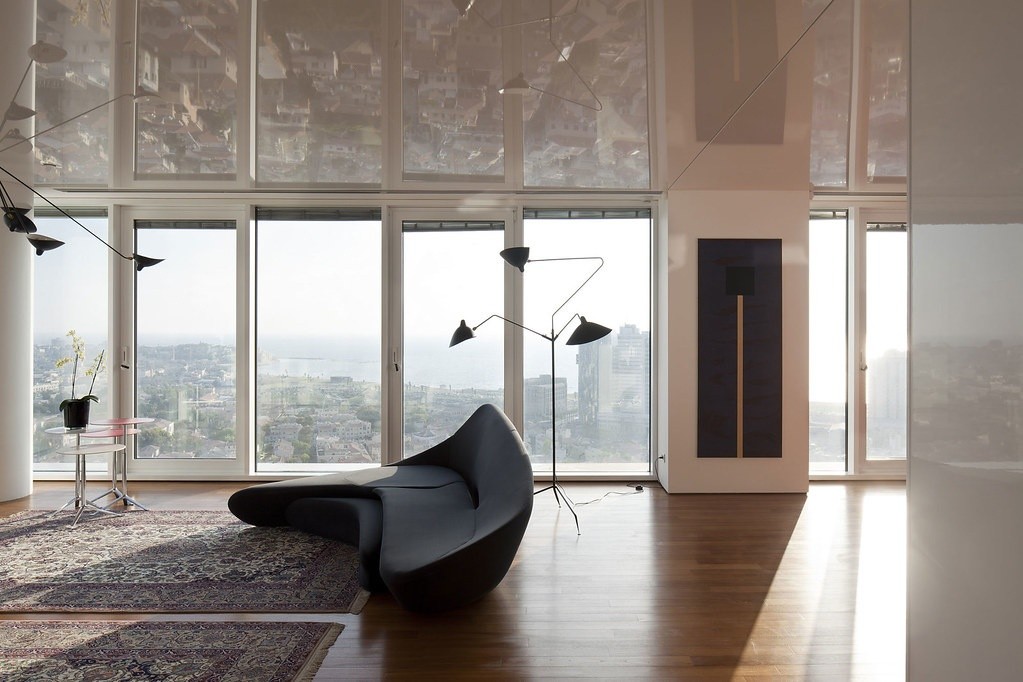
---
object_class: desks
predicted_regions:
[79,429,141,507]
[88,417,156,515]
[56,444,126,526]
[45,425,111,520]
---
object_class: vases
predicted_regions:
[63,400,90,428]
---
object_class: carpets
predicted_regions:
[0,509,369,614]
[0,619,344,682]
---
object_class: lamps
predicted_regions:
[0,166,166,272]
[448,247,613,536]
[455,0,603,113]
[0,40,165,153]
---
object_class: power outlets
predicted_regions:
[661,453,664,461]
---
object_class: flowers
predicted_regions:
[56,329,107,413]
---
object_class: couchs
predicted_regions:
[228,403,535,613]
